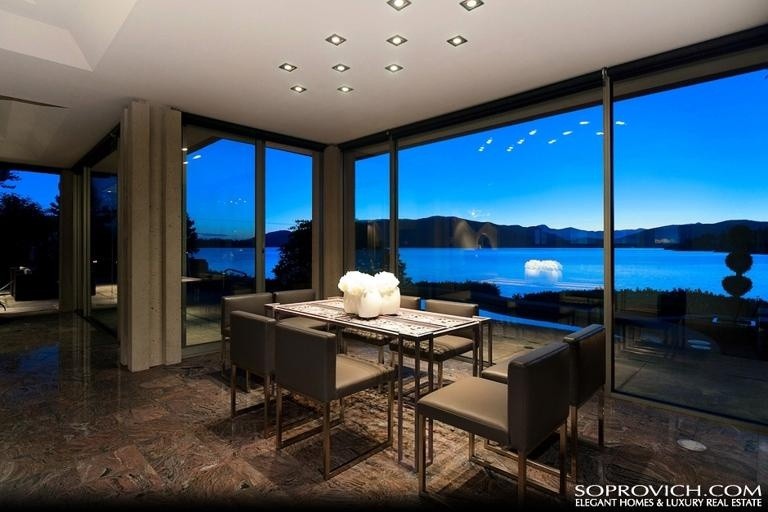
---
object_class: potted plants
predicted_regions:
[709,223,758,352]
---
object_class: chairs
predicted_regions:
[220,287,689,512]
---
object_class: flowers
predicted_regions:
[337,270,401,296]
